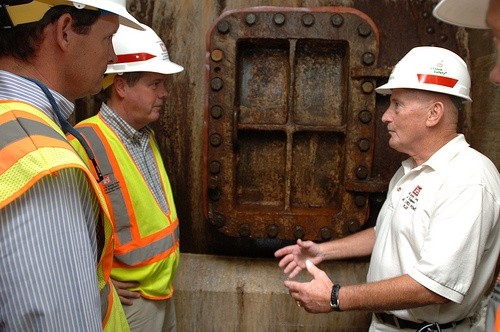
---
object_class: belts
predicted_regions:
[375,310,465,331]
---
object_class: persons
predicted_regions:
[1,0,146,331]
[64,23,184,332]
[432,1,500,88]
[274,45,500,331]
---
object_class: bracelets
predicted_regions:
[329,284,343,313]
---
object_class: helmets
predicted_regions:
[375,46,473,103]
[65,0,185,74]
